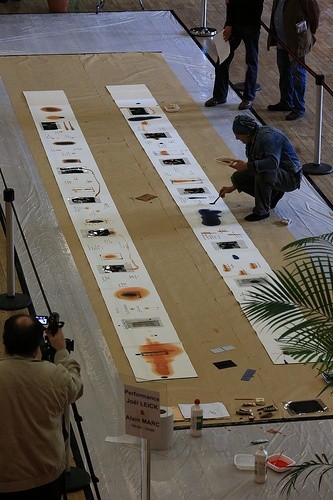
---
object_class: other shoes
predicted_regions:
[252,206,273,213]
[267,102,293,111]
[244,213,270,221]
[205,98,226,107]
[238,99,253,110]
[286,112,304,121]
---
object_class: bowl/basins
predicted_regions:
[267,453,296,473]
[233,453,255,471]
[215,156,234,166]
[164,104,180,112]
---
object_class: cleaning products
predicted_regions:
[250,439,269,484]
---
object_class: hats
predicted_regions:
[233,115,257,135]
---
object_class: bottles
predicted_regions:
[191,399,203,437]
[254,445,268,483]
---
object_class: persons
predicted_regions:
[267,0,321,121]
[219,115,303,221]
[0,314,83,500]
[205,0,265,110]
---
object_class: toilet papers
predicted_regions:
[149,405,173,451]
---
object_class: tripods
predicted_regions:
[64,402,102,500]
